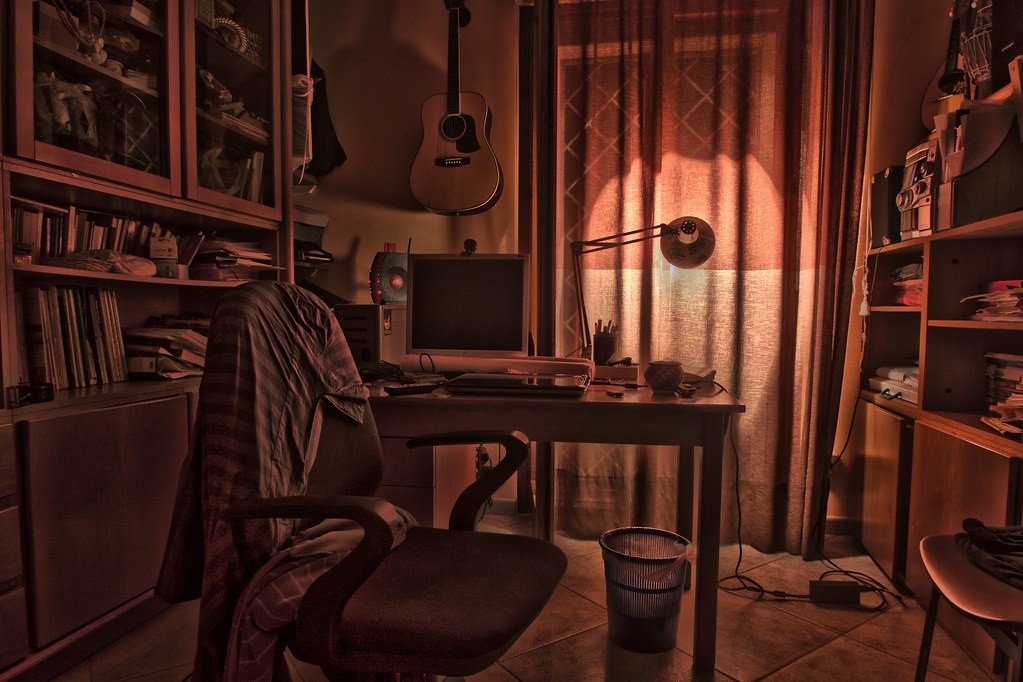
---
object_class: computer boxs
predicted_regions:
[333,302,406,380]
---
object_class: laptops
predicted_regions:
[443,372,590,397]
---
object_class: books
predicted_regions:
[8,205,287,392]
[960,280,1023,438]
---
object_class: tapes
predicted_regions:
[154,261,190,282]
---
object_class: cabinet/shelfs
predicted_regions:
[852,74,1023,680]
[1,1,297,682]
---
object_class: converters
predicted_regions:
[808,579,861,606]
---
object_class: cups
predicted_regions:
[593,332,618,364]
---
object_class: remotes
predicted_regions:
[384,382,439,395]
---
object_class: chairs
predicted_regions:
[203,278,569,676]
[911,517,1022,682]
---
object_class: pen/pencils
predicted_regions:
[593,319,618,335]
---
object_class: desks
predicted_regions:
[345,369,746,680]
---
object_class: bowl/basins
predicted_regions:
[644,361,685,395]
[28,383,54,402]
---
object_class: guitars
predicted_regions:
[409,0,504,217]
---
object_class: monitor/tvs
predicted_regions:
[405,252,529,360]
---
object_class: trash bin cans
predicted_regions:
[599,525,693,655]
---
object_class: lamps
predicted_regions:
[571,215,716,382]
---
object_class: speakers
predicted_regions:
[870,165,906,248]
[369,252,408,303]
